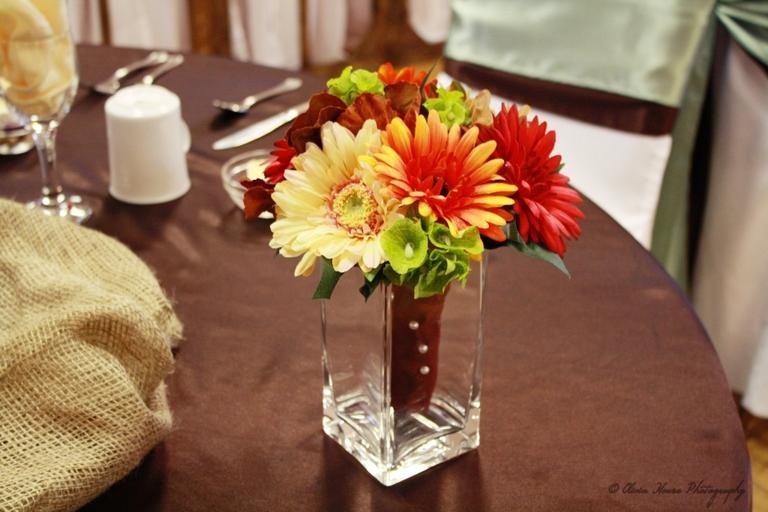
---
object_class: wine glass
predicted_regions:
[0,31,94,226]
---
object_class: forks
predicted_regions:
[211,76,304,113]
[77,49,185,94]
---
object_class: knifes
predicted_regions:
[212,101,310,152]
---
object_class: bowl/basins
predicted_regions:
[220,148,276,219]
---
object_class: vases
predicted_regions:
[318,249,483,487]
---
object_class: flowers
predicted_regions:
[238,63,589,418]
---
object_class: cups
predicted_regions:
[104,84,192,206]
